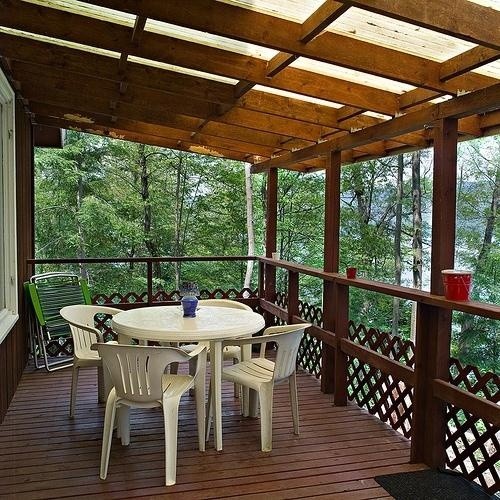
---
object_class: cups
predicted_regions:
[272,253,280,262]
[346,268,357,279]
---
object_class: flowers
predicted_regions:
[181,280,200,296]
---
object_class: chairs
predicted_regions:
[206,323,313,452]
[24,272,92,372]
[189,299,253,396]
[90,344,207,486]
[59,304,124,421]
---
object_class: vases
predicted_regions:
[181,293,198,318]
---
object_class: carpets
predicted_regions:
[374,466,499,500]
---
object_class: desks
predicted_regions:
[112,306,266,452]
